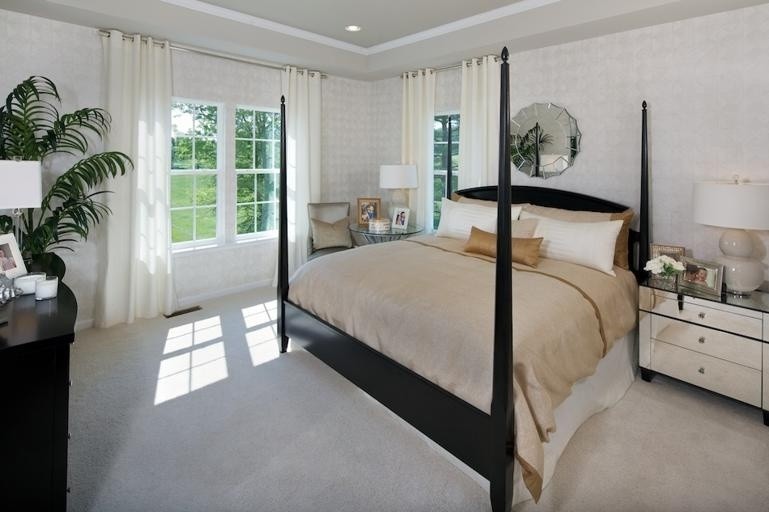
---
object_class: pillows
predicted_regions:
[522,201,636,273]
[496,213,540,240]
[431,196,524,240]
[516,207,624,278]
[449,190,533,212]
[462,223,545,270]
[307,216,356,251]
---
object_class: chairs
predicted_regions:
[302,201,360,264]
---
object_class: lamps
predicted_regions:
[686,172,769,300]
[379,162,420,222]
[0,154,44,248]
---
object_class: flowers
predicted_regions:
[639,254,687,282]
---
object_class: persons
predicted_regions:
[0,248,15,269]
[366,202,376,219]
[685,265,697,281]
[694,267,709,286]
[400,211,405,225]
[396,214,402,225]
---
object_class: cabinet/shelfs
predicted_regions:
[1,247,89,510]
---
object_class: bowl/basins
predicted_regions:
[12,272,47,296]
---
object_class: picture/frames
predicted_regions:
[674,253,725,303]
[0,230,27,284]
[391,207,413,230]
[355,196,382,227]
[647,243,687,282]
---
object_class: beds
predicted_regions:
[268,45,656,512]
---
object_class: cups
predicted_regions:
[36,275,59,302]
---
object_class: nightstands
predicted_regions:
[347,218,426,244]
[632,268,769,429]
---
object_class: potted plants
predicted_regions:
[0,74,139,286]
